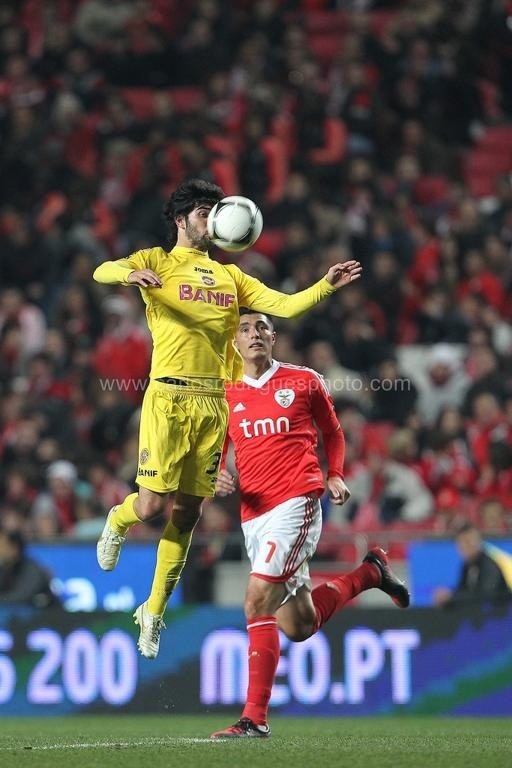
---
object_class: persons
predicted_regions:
[90,177,362,656]
[183,503,228,606]
[213,307,413,739]
[430,522,511,606]
[1,527,66,608]
[0,0,512,535]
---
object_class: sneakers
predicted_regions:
[133,599,167,660]
[211,717,271,738]
[362,547,409,609]
[96,504,130,572]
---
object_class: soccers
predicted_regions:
[205,194,264,255]
